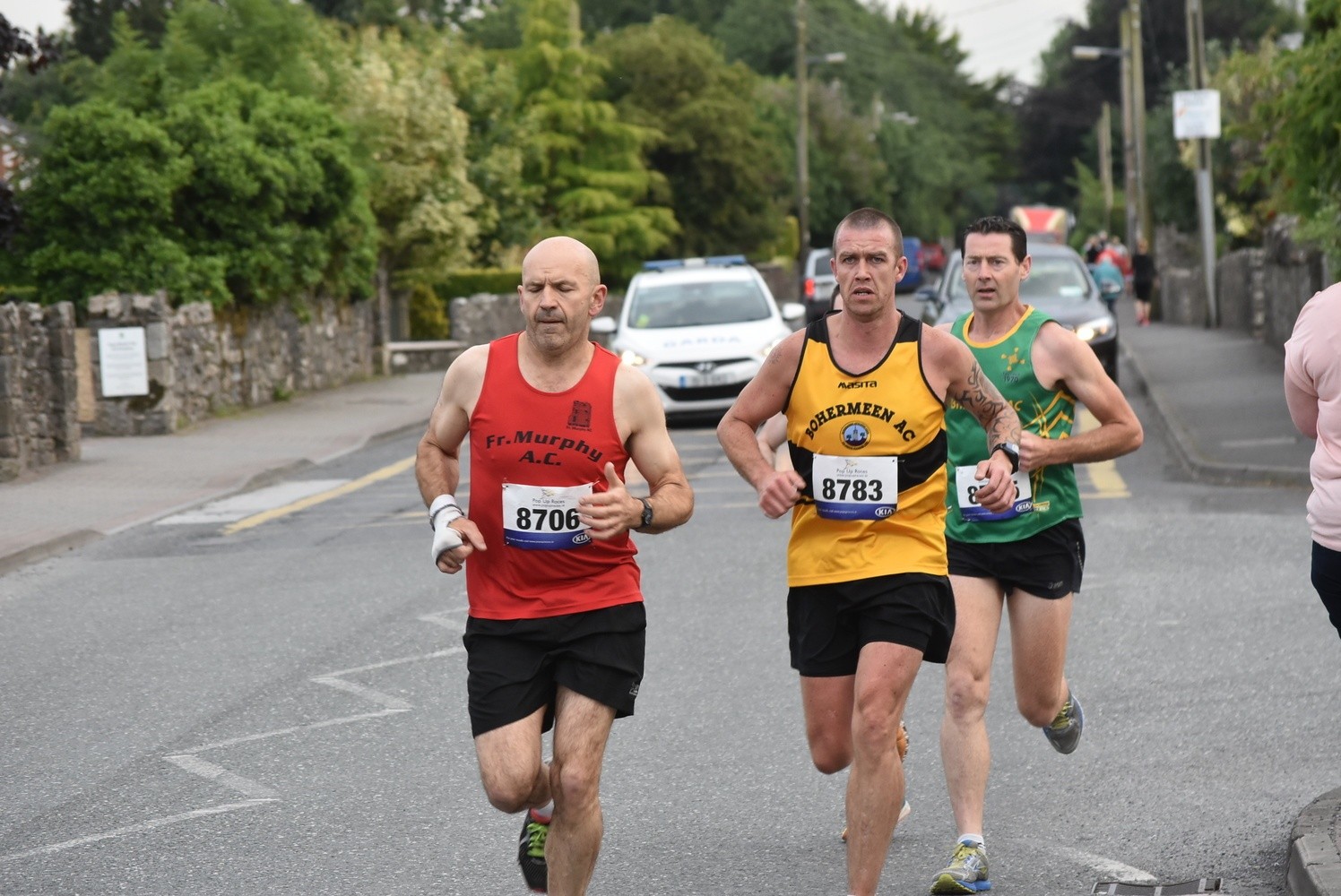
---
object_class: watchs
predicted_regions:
[633,497,653,532]
[991,442,1021,476]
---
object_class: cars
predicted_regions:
[894,237,928,295]
[922,243,949,274]
[920,241,1125,389]
[590,254,808,428]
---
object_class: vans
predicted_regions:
[800,249,842,323]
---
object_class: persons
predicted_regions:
[1282,278,1341,642]
[927,216,1145,896]
[415,236,696,895]
[1080,226,1157,329]
[716,207,1025,896]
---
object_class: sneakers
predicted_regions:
[517,806,553,891]
[1041,691,1084,754]
[930,838,991,893]
[842,798,911,842]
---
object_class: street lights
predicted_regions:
[794,52,844,256]
[1071,44,1144,307]
